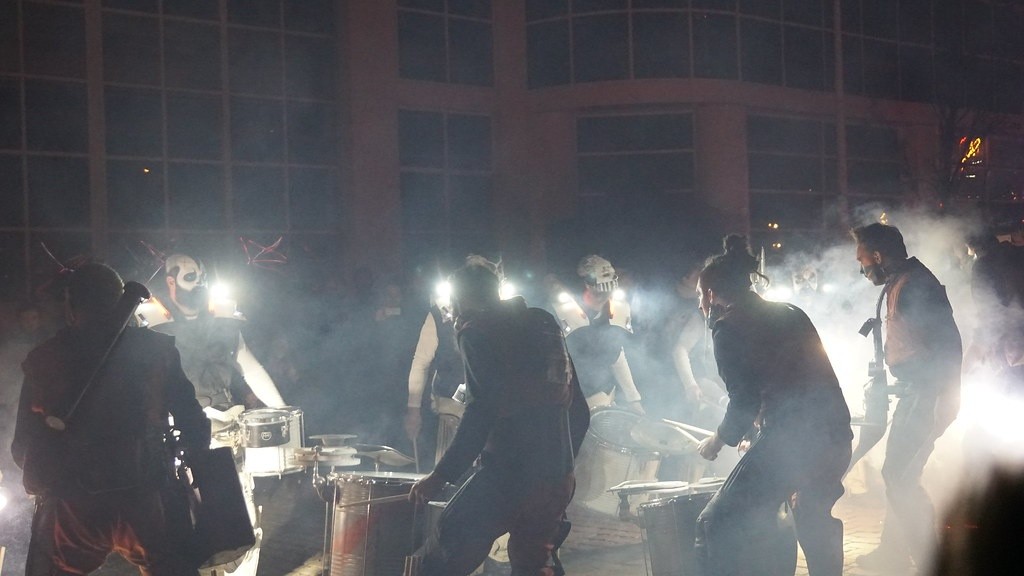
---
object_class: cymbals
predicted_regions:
[290,428,415,473]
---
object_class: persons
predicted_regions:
[669,302,732,433]
[695,230,854,576]
[131,253,288,444]
[548,253,648,418]
[409,264,591,576]
[848,222,962,575]
[402,255,505,469]
[781,250,837,307]
[11,263,213,576]
[963,227,1024,393]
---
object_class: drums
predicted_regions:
[238,405,306,478]
[638,490,799,576]
[176,446,257,569]
[577,405,668,520]
[329,471,434,576]
